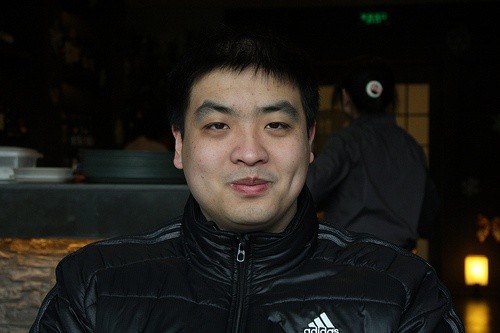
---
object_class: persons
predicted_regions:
[28,35,466,333]
[301,54,442,253]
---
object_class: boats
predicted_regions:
[0,144,192,240]
